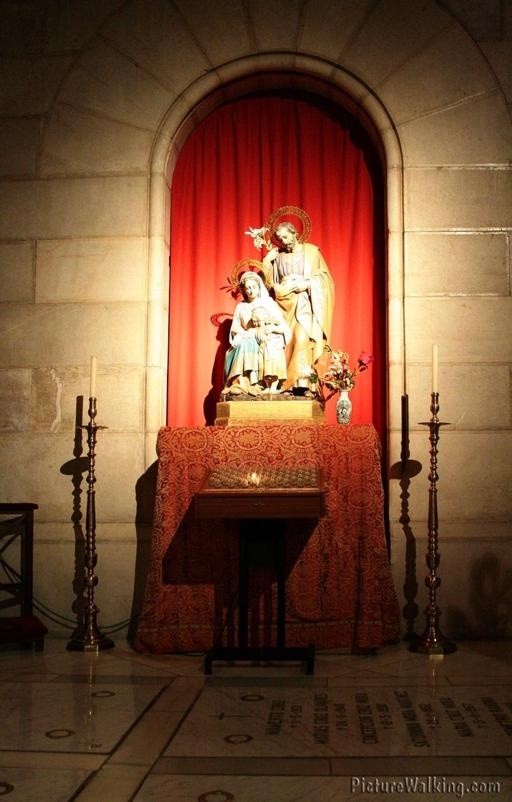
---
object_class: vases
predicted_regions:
[336,390,352,425]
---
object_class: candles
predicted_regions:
[88,356,95,397]
[432,345,439,395]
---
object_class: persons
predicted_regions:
[224,272,292,396]
[253,222,335,397]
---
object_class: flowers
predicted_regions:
[304,343,375,393]
